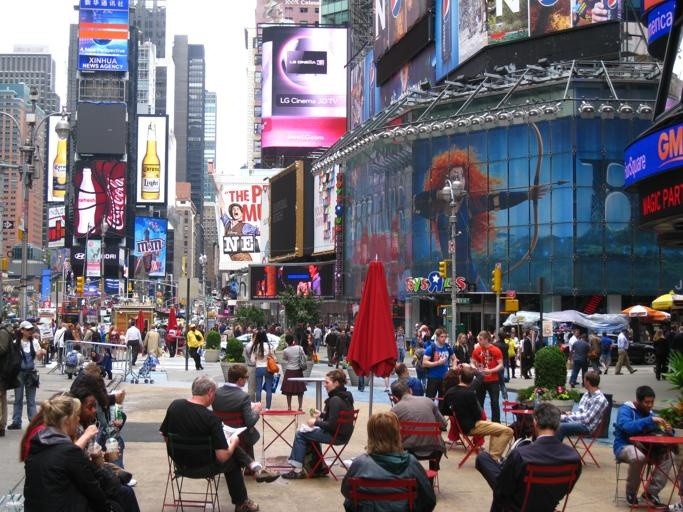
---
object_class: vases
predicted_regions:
[346,366,370,388]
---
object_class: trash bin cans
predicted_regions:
[579,393,612,438]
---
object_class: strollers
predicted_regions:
[129,353,160,384]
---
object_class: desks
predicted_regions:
[258,409,305,470]
[285,377,326,412]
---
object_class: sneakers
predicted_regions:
[282,470,305,479]
[642,492,683,512]
[235,500,259,512]
[254,467,279,482]
[626,493,638,507]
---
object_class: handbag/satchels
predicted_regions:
[54,343,58,351]
[266,356,279,373]
[19,320,34,329]
[298,352,306,370]
[262,374,280,392]
[588,350,596,359]
[195,335,201,341]
[25,372,40,388]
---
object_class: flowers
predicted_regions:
[516,383,581,403]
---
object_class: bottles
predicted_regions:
[114,404,122,423]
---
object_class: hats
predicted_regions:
[190,323,195,327]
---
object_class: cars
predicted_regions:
[219,333,287,360]
[559,333,655,365]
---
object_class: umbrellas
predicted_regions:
[346,261,396,418]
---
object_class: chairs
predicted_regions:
[348,475,419,512]
[160,432,223,512]
[211,410,256,476]
[387,391,683,512]
[304,408,360,481]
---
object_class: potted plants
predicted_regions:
[202,329,221,363]
[218,335,249,383]
[654,347,683,479]
[278,282,324,387]
[273,338,287,363]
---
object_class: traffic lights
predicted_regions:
[98,283,103,292]
[76,277,84,294]
[439,307,448,318]
[438,260,447,279]
[491,268,502,293]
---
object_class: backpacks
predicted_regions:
[416,344,451,378]
[1,326,21,379]
[90,328,100,343]
[66,351,79,366]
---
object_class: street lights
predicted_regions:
[199,253,208,297]
[99,215,109,283]
[441,179,463,344]
[0,86,72,324]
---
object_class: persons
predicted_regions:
[474,402,583,511]
[339,409,437,511]
[158,373,260,511]
[412,144,552,291]
[590,1,609,25]
[256,272,268,297]
[218,185,260,262]
[21,395,140,511]
[0,290,682,486]
[294,265,323,297]
[613,385,678,508]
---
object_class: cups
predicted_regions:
[105,438,120,461]
[85,443,103,463]
